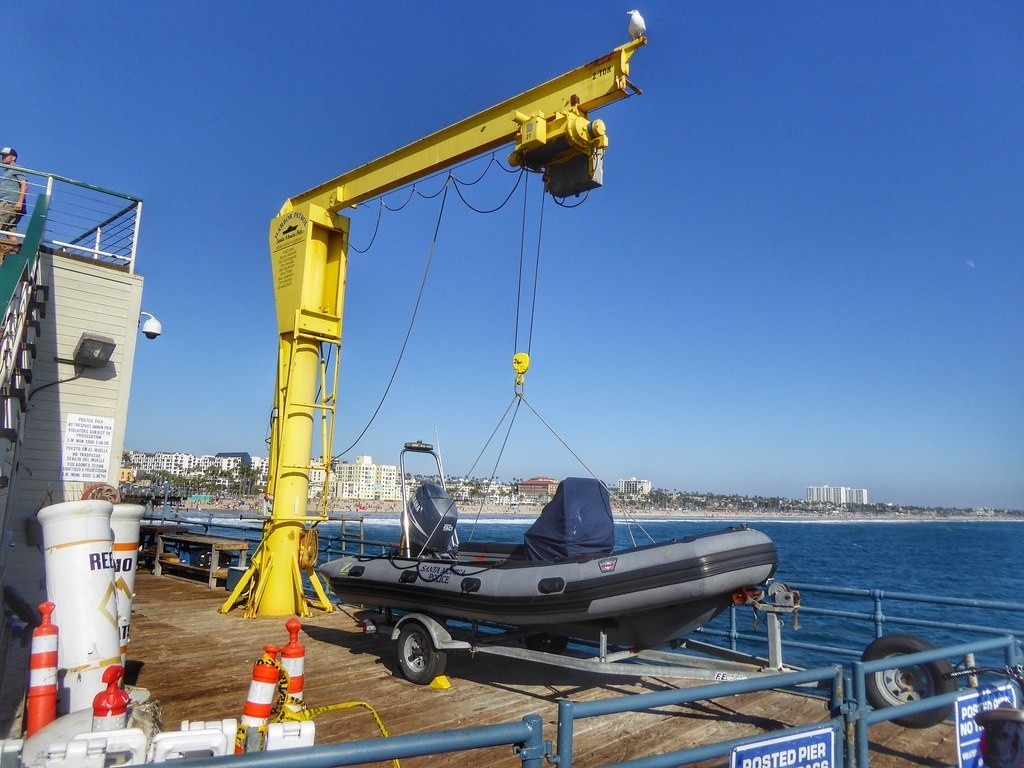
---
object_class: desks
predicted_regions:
[154,533,249,589]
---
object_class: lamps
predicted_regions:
[53,332,116,367]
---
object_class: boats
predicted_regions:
[318,440,778,648]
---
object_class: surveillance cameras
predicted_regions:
[142,318,161,339]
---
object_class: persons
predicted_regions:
[0,147,28,241]
[974,702,1024,768]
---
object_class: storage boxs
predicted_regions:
[160,546,202,565]
[226,566,250,592]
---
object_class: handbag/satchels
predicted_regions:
[21,198,27,214]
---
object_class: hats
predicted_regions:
[0,147,17,157]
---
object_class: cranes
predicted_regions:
[211,30,648,620]
